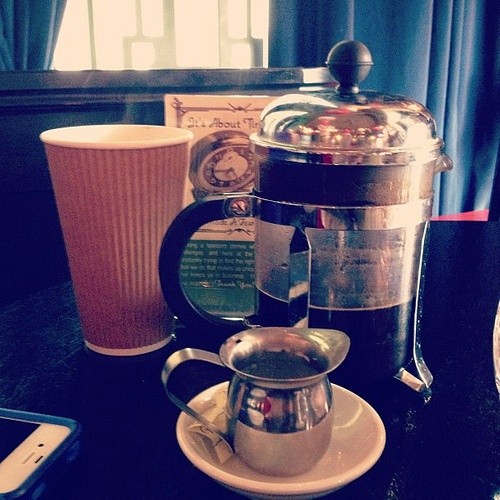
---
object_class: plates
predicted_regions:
[176,380,386,500]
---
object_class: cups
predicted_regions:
[162,328,352,475]
[39,123,194,356]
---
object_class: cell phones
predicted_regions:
[0,407,80,500]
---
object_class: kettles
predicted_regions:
[159,38,451,402]
